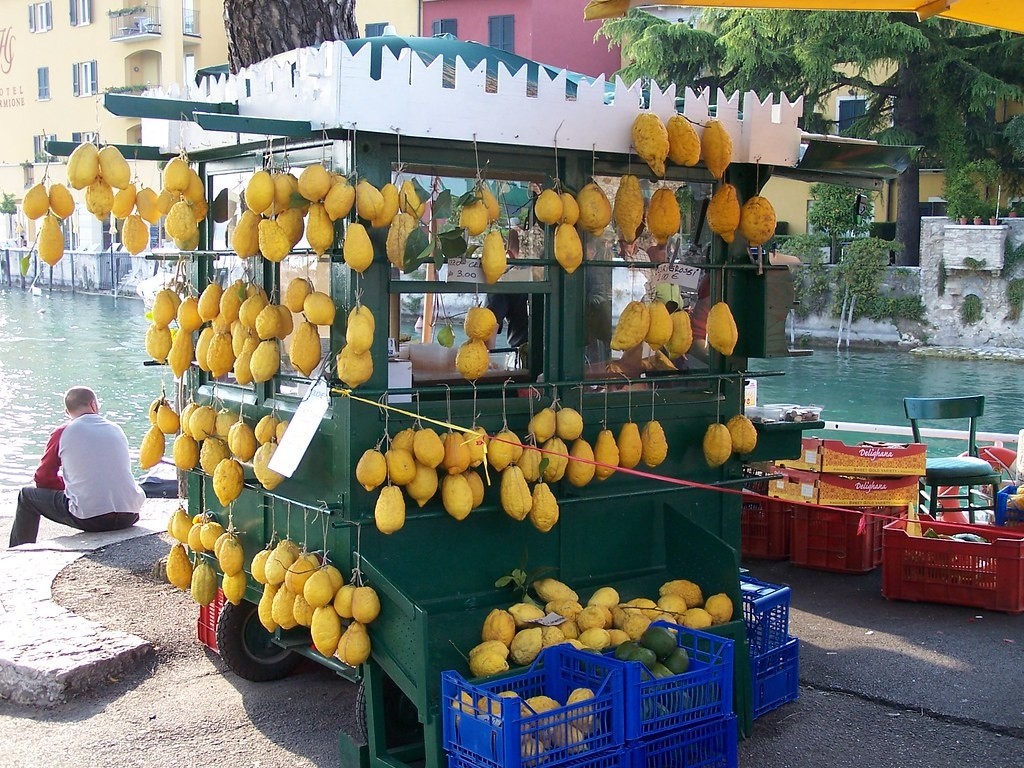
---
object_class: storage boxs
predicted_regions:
[196,586,227,655]
[740,437,1024,613]
[749,636,799,719]
[446,747,631,767]
[442,645,624,767]
[559,619,734,740]
[628,714,738,767]
[740,574,792,655]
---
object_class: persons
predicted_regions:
[584,237,710,372]
[7,386,146,548]
[478,226,544,382]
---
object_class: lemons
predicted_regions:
[145,279,375,391]
[356,404,669,535]
[137,397,293,507]
[467,577,733,715]
[23,144,207,267]
[452,685,598,768]
[703,414,757,467]
[251,539,381,666]
[232,165,424,272]
[164,507,246,607]
[438,307,498,381]
[459,114,777,283]
[610,302,738,359]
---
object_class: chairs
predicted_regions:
[904,395,1002,523]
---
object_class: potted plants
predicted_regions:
[959,206,1017,225]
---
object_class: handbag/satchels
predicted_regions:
[138,476,178,498]
